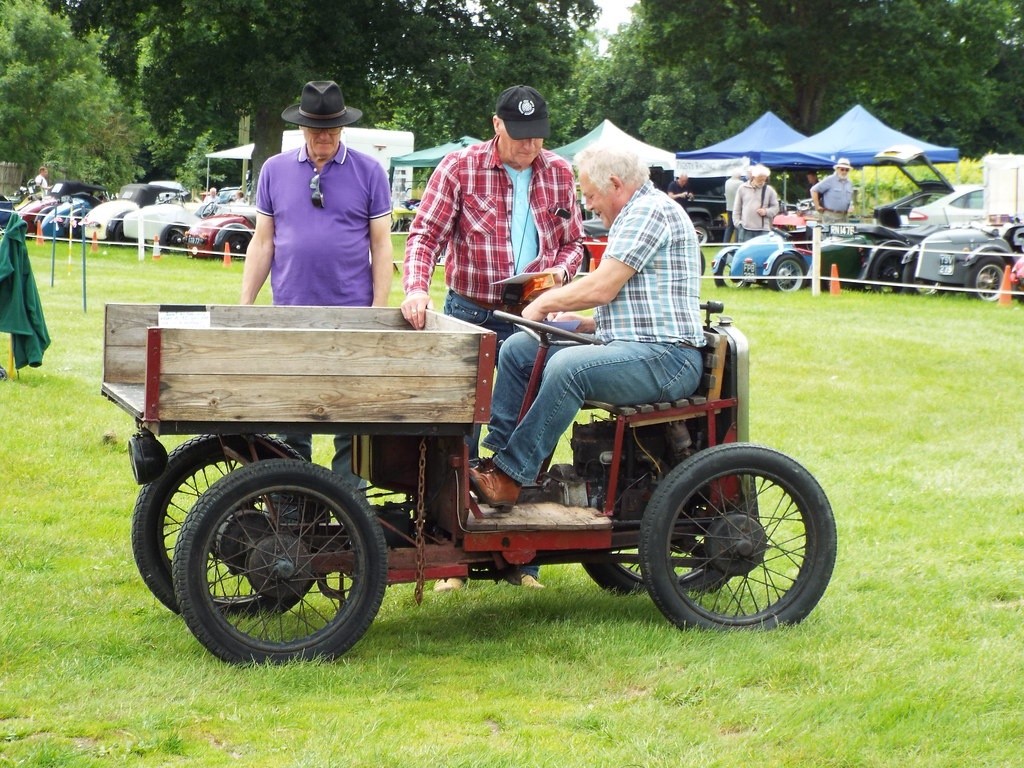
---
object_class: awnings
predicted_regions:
[206,138,305,191]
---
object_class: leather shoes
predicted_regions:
[467,457,521,514]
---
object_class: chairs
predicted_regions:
[537,331,728,517]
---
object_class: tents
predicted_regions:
[549,119,676,177]
[761,104,960,210]
[674,111,865,217]
[389,135,481,193]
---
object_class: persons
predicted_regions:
[399,85,585,592]
[236,192,245,203]
[723,169,744,243]
[239,82,394,524]
[667,173,693,211]
[204,187,219,204]
[470,142,706,513]
[733,164,779,286]
[809,158,853,223]
[806,170,820,198]
[36,167,53,195]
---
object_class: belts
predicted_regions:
[457,293,530,316]
[826,208,846,216]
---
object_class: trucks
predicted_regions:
[99,298,838,668]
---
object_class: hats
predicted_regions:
[496,85,551,140]
[280,80,364,128]
[834,158,853,170]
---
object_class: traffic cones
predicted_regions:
[997,265,1012,305]
[830,264,840,295]
[90,230,99,253]
[223,242,231,267]
[152,235,160,260]
[36,220,44,245]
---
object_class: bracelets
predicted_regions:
[564,275,567,284]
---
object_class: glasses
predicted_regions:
[309,174,325,208]
[308,127,343,135]
[841,168,850,172]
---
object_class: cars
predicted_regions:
[676,155,1024,302]
[0,181,257,260]
